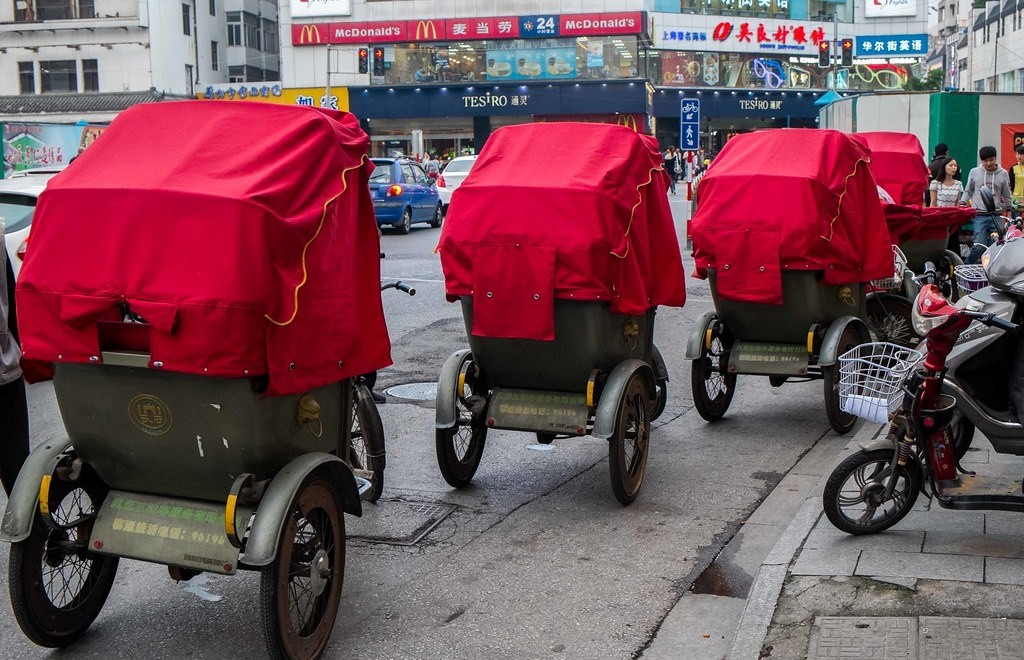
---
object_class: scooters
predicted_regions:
[821,190,1024,537]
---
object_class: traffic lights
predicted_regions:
[817,40,832,69]
[374,48,385,76]
[358,46,369,74]
[840,38,853,67]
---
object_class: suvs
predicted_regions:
[0,162,70,283]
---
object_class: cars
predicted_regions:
[367,155,444,234]
[435,154,481,215]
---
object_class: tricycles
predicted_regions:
[685,127,972,437]
[433,124,688,506]
[1,99,419,660]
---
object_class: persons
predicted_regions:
[1009,146,1024,219]
[929,143,961,180]
[453,67,475,81]
[959,146,1012,264]
[69,147,84,165]
[415,67,426,82]
[928,157,964,255]
[662,145,709,196]
[421,150,471,179]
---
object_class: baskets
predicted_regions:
[838,342,922,423]
[871,245,907,290]
[954,264,989,297]
